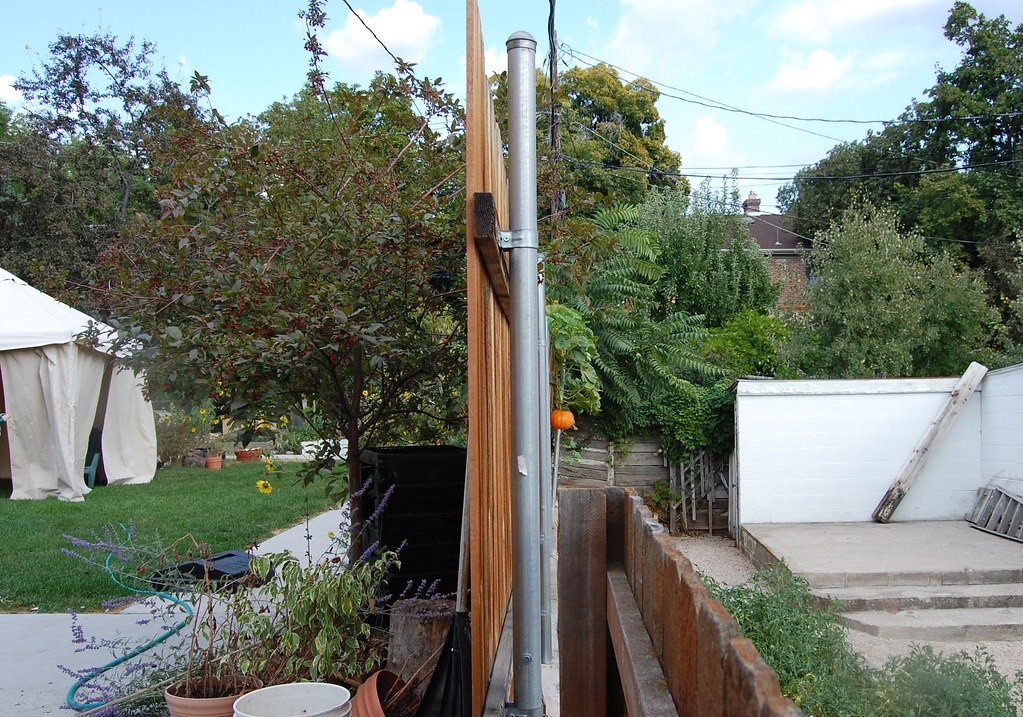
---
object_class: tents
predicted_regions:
[0,264,158,504]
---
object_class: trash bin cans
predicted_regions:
[360,443,471,641]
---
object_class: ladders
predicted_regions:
[968,480,1023,543]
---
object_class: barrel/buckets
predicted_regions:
[232,682,352,717]
[206,457,221,469]
[351,669,418,717]
[235,448,262,462]
[165,674,263,717]
